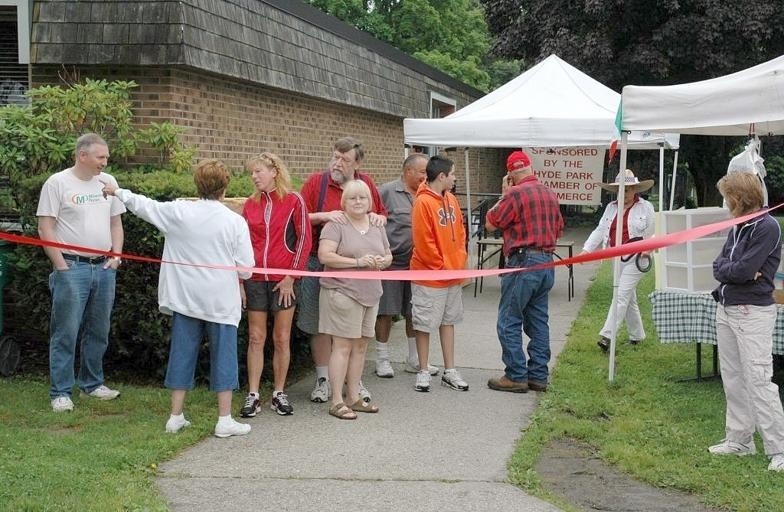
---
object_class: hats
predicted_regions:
[594,169,655,194]
[505,150,531,172]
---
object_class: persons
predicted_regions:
[100,159,256,437]
[316,180,395,420]
[238,151,314,416]
[708,171,784,472]
[408,154,469,392]
[484,150,563,393]
[577,168,655,352]
[377,153,440,378]
[295,138,389,404]
[35,133,127,413]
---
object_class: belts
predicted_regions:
[62,254,108,264]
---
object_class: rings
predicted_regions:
[369,261,371,263]
[369,257,373,261]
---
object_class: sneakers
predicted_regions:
[79,384,121,401]
[375,356,469,391]
[708,438,757,456]
[310,376,332,402]
[597,337,611,354]
[214,420,251,438]
[164,413,191,434]
[49,397,75,413]
[527,378,547,391]
[344,378,372,402]
[767,453,784,473]
[487,375,530,393]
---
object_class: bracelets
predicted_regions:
[498,195,505,200]
[111,254,122,264]
[356,258,359,269]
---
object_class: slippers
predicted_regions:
[349,400,379,413]
[329,402,357,420]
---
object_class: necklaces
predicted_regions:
[348,214,368,236]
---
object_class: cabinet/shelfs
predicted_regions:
[653,209,734,293]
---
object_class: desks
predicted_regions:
[650,286,784,382]
[474,240,575,302]
